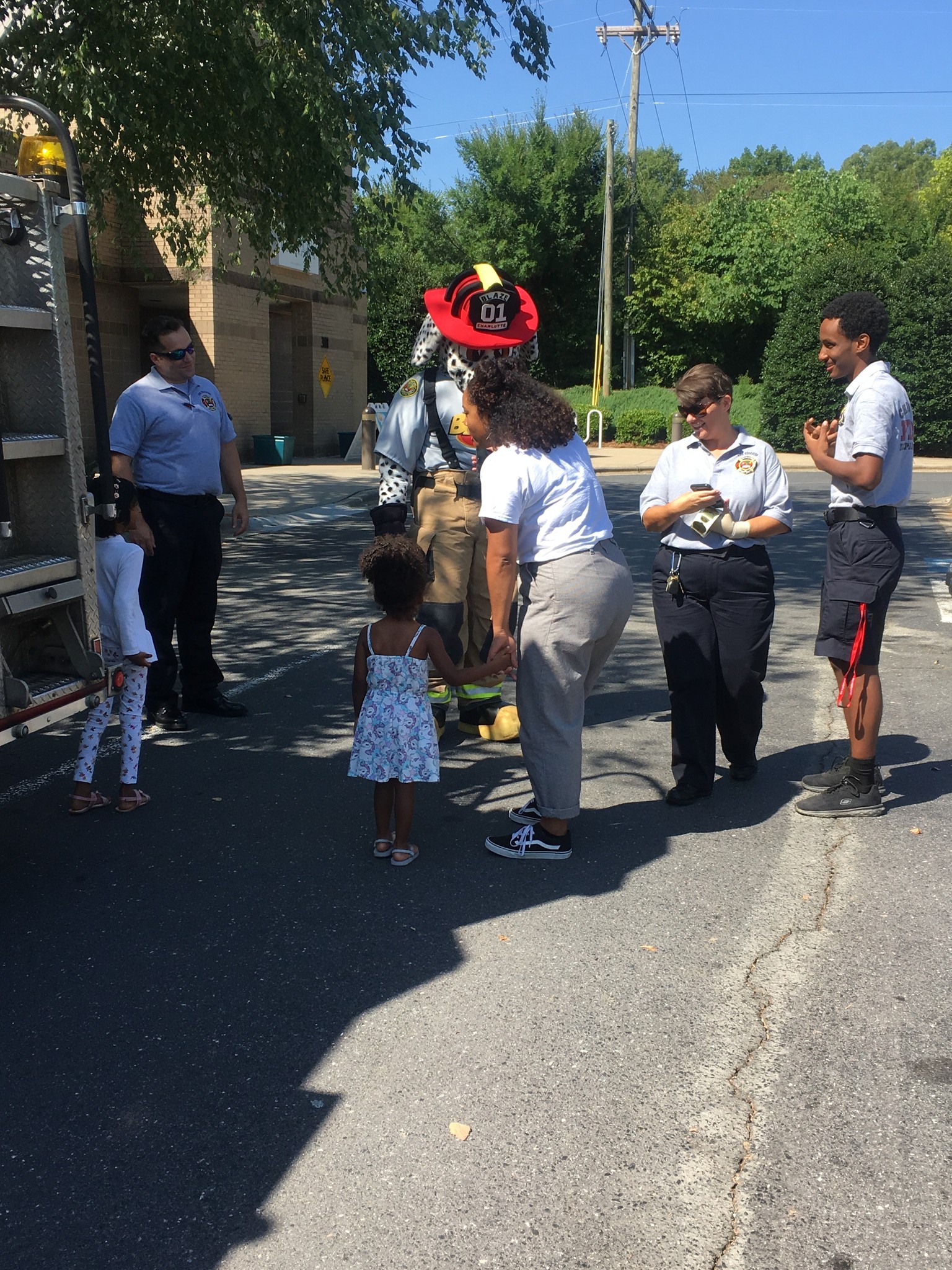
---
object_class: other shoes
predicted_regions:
[458,698,524,741]
[666,783,714,807]
[728,756,757,781]
[433,707,447,740]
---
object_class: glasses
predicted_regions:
[151,341,195,361]
[676,394,720,418]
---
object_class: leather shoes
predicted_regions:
[147,703,190,730]
[184,694,248,717]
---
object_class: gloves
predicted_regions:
[369,501,409,536]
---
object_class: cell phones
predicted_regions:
[691,483,724,508]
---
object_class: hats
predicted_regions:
[423,264,539,350]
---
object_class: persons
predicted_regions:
[794,291,913,818]
[462,356,634,862]
[69,469,159,814]
[109,316,250,729]
[640,363,793,807]
[352,534,513,866]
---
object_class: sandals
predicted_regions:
[115,789,151,813]
[374,839,393,857]
[391,844,420,866]
[68,791,113,814]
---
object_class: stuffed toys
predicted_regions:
[371,263,538,737]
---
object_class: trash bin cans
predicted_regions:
[252,435,296,464]
[335,431,359,455]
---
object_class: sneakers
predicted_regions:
[485,824,573,860]
[794,775,886,818]
[509,798,544,824]
[801,753,886,793]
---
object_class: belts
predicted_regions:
[823,507,900,526]
[417,476,479,499]
